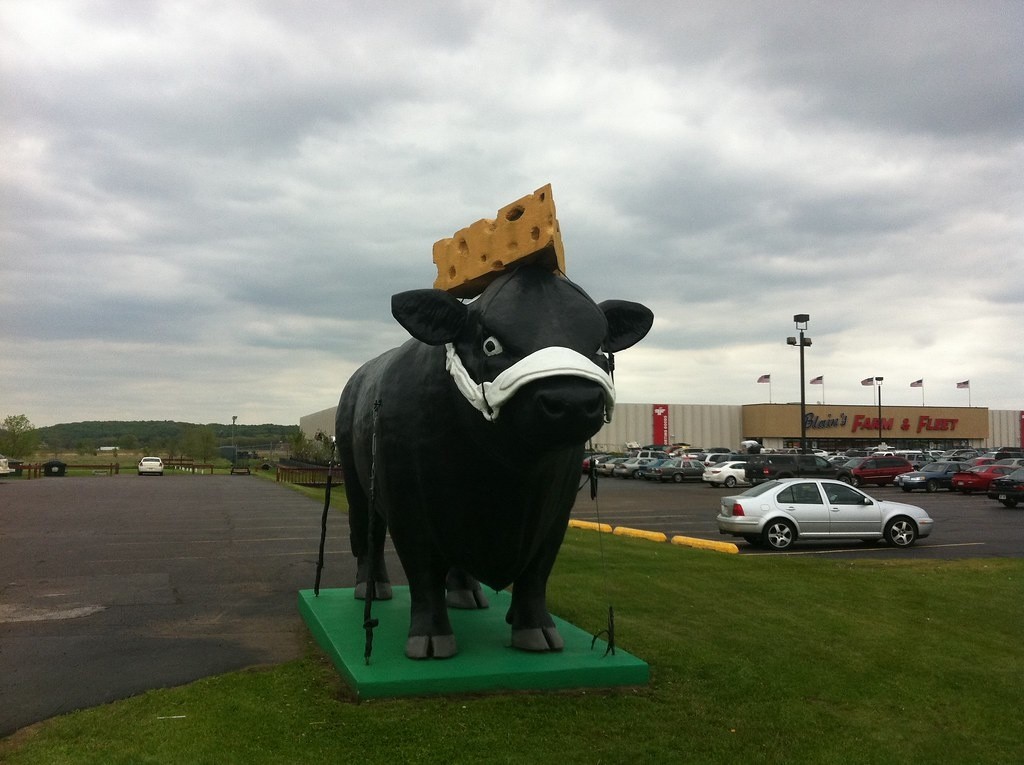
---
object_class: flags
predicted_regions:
[810,376,822,384]
[757,374,770,383]
[910,379,922,387]
[957,381,968,388]
[861,378,873,385]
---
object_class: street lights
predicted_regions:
[232,416,238,467]
[875,376,883,444]
[787,314,812,453]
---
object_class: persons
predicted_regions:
[913,464,920,471]
[830,450,839,455]
[742,445,746,454]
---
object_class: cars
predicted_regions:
[582,439,1024,507]
[717,476,933,549]
[0,454,15,474]
[138,457,164,476]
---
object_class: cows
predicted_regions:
[335,262,653,660]
